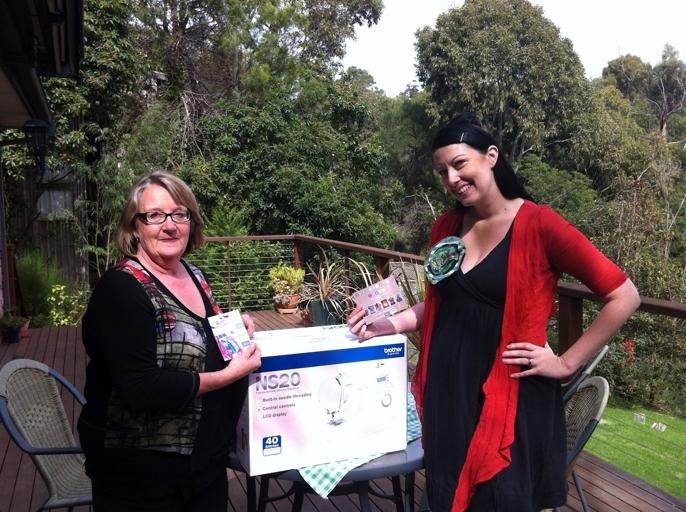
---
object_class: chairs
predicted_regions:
[554,344,610,403]
[417,375,610,511]
[0,358,92,512]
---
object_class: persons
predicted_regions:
[349,125,641,512]
[77,170,262,512]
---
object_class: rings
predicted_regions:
[527,359,532,367]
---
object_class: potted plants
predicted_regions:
[269,266,305,315]
[294,252,357,326]
[0,316,29,343]
[297,295,312,323]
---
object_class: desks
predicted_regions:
[223,381,426,512]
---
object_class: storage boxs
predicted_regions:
[234,323,407,478]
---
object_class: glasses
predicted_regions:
[134,211,191,223]
